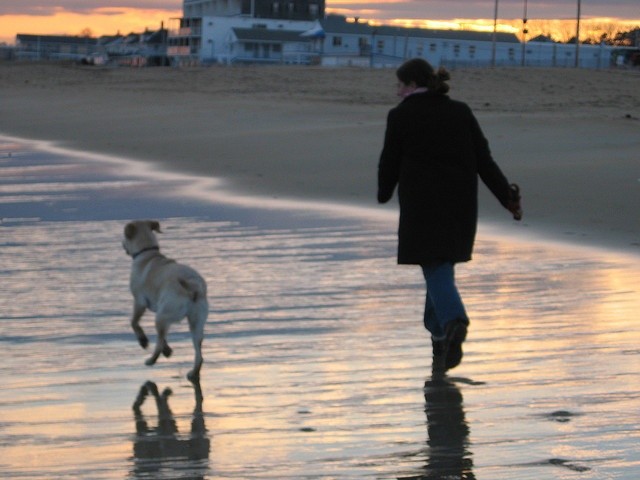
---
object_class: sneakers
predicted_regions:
[445,320,467,372]
[431,339,450,358]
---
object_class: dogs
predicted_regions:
[122,219,208,381]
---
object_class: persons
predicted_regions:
[377,58,522,369]
